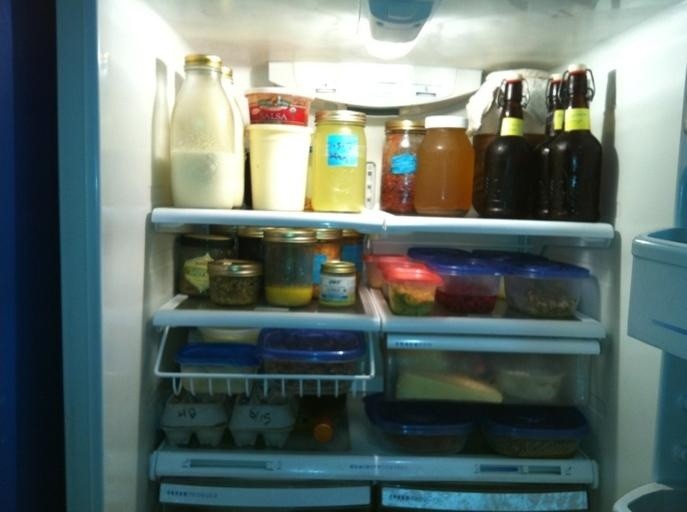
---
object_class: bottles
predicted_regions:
[471,67,551,217]
[537,77,569,217]
[479,75,538,217]
[244,87,312,123]
[247,124,313,212]
[169,53,236,210]
[220,67,246,208]
[548,63,602,221]
[177,226,366,308]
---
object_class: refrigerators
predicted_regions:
[59,0,687,512]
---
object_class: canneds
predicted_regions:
[178,224,364,309]
